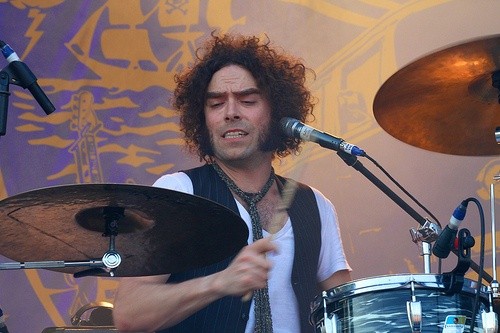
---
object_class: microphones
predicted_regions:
[278,115,366,157]
[433,199,468,259]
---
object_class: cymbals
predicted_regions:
[373,34,500,157]
[0,184,250,276]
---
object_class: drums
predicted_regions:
[308,273,492,333]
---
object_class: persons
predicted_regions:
[113,28,355,333]
